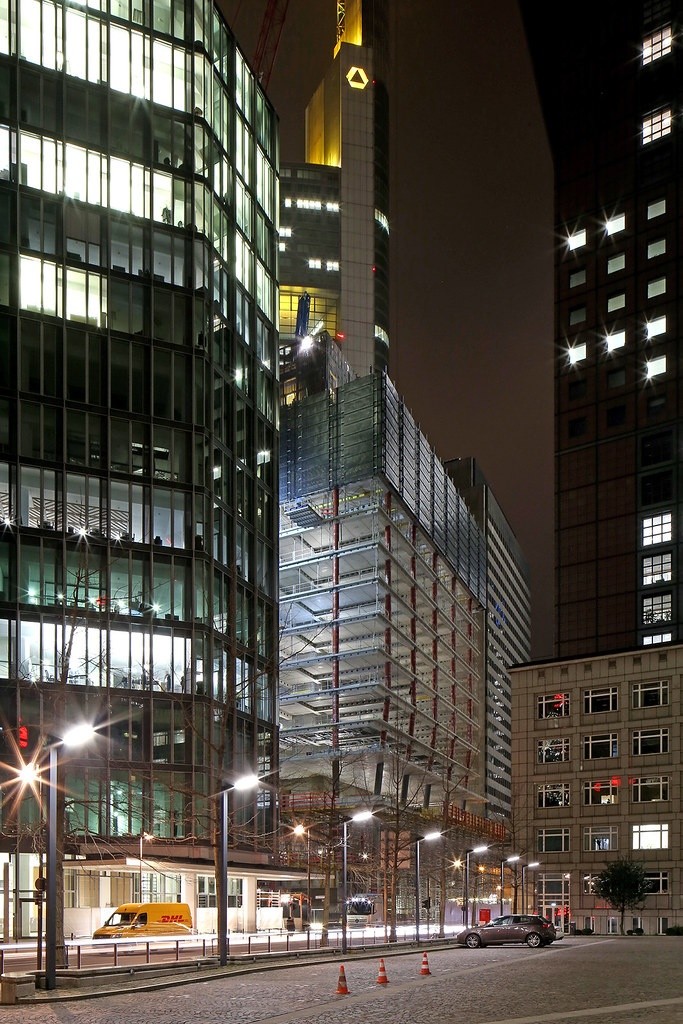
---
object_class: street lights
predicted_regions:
[466,847,486,929]
[415,832,441,946]
[214,774,259,966]
[501,854,520,916]
[18,723,93,991]
[341,811,375,950]
[294,825,311,934]
[522,863,539,913]
[138,831,153,903]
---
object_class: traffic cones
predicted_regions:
[419,952,431,975]
[377,959,389,983]
[334,965,352,994]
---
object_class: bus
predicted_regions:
[346,899,376,930]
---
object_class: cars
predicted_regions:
[457,914,556,948]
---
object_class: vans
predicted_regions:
[93,902,193,939]
